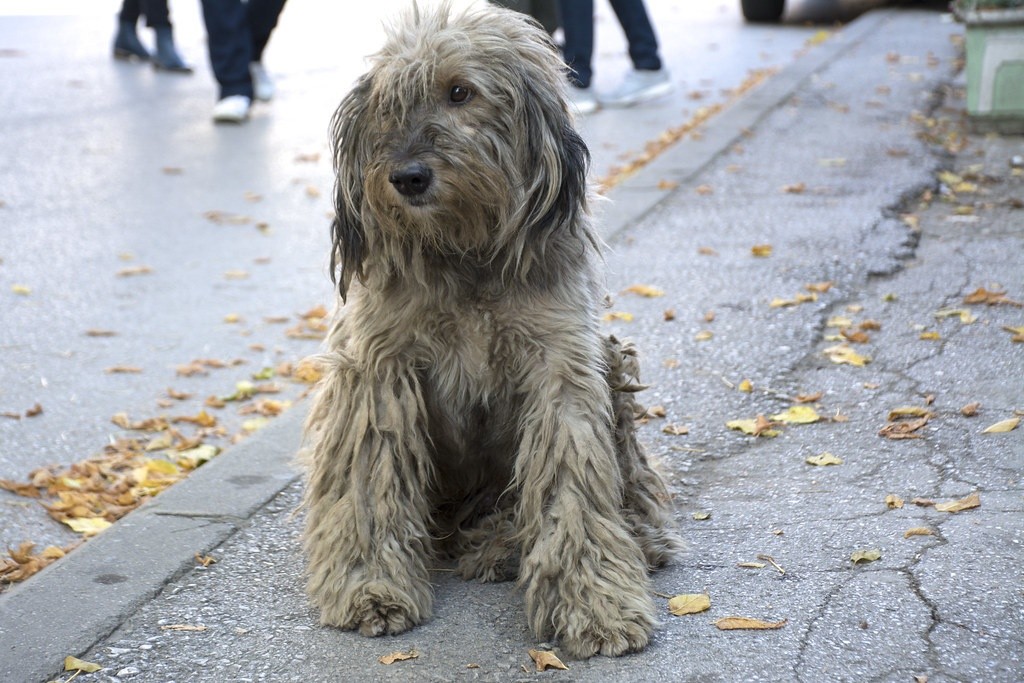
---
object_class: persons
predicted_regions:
[113,0,194,74]
[199,0,286,120]
[488,0,671,112]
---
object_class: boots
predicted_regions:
[115,21,150,61]
[153,25,191,73]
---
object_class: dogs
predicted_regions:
[292,0,691,663]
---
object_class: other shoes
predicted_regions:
[602,59,672,101]
[212,95,251,123]
[248,60,273,101]
[561,81,597,115]
